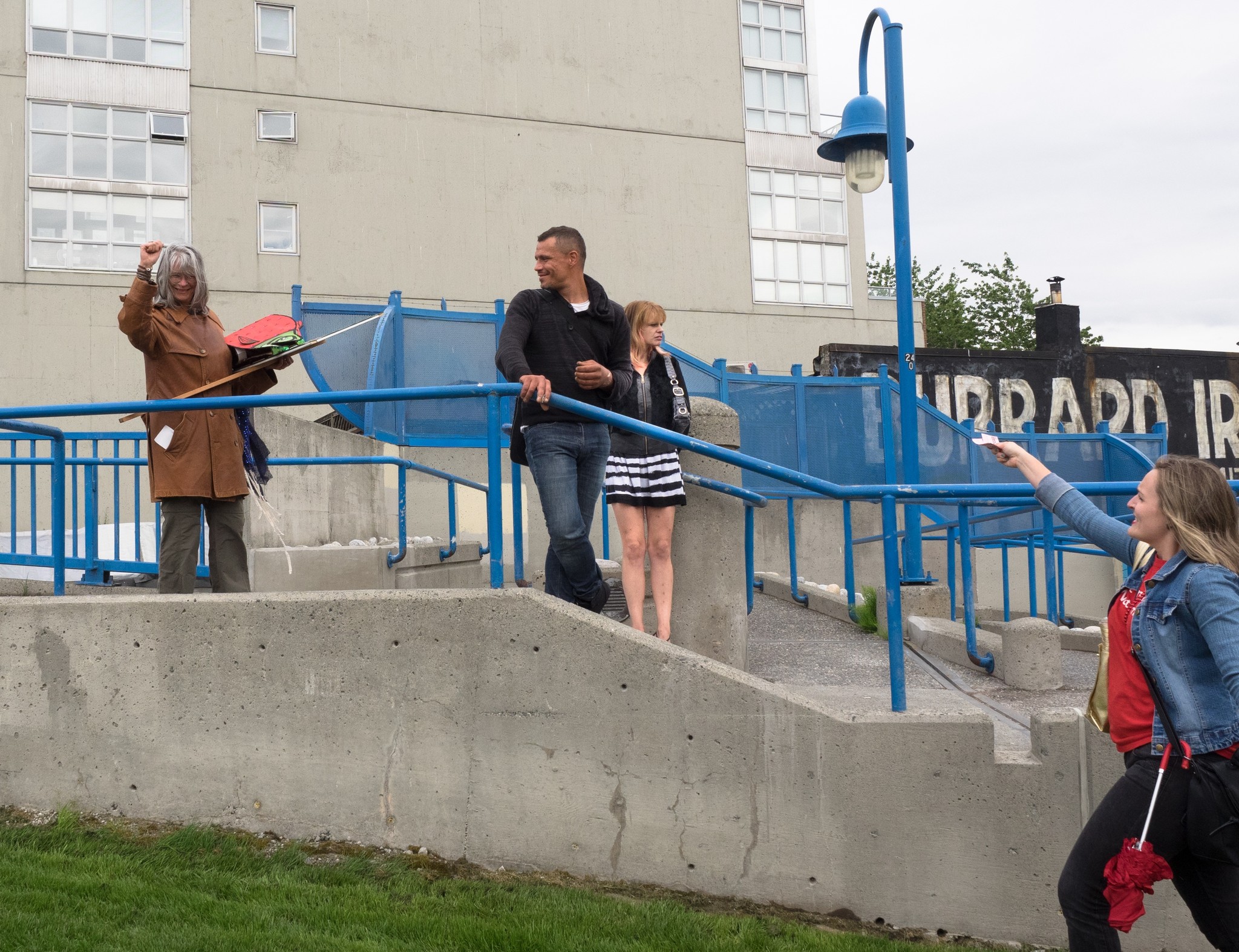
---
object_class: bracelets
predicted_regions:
[136,264,152,281]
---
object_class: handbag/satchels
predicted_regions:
[664,353,690,433]
[1184,756,1239,863]
[508,395,529,467]
[1085,540,1158,732]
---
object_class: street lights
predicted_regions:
[817,7,939,584]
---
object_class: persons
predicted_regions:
[494,225,633,617]
[118,240,296,597]
[987,436,1239,952]
[604,299,692,645]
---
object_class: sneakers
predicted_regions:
[576,581,611,614]
